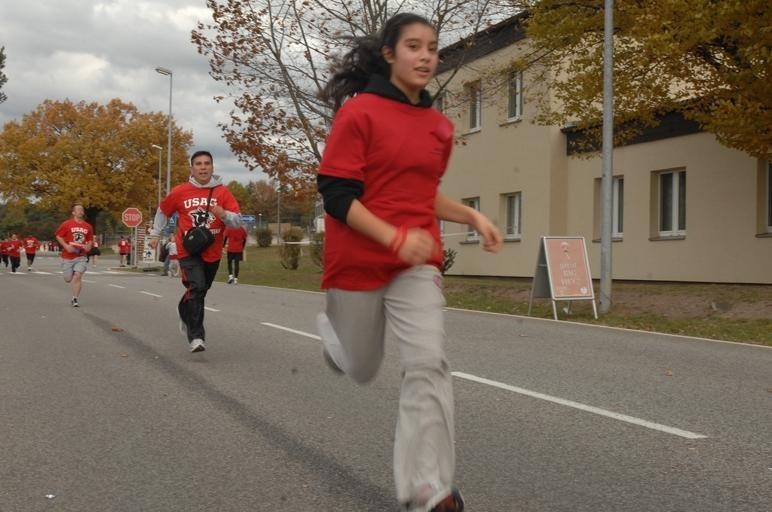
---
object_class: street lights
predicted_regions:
[152,67,173,207]
[259,214,263,227]
[275,185,291,246]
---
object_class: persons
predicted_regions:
[148,150,244,353]
[55,201,94,307]
[0,232,182,277]
[312,12,503,510]
[221,224,248,286]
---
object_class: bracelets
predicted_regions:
[388,221,410,258]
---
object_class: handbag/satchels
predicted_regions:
[182,225,214,256]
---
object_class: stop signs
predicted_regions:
[122,208,142,227]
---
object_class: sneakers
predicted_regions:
[318,312,346,376]
[71,296,79,308]
[187,337,206,353]
[227,274,239,285]
[175,304,188,336]
[405,490,465,512]
